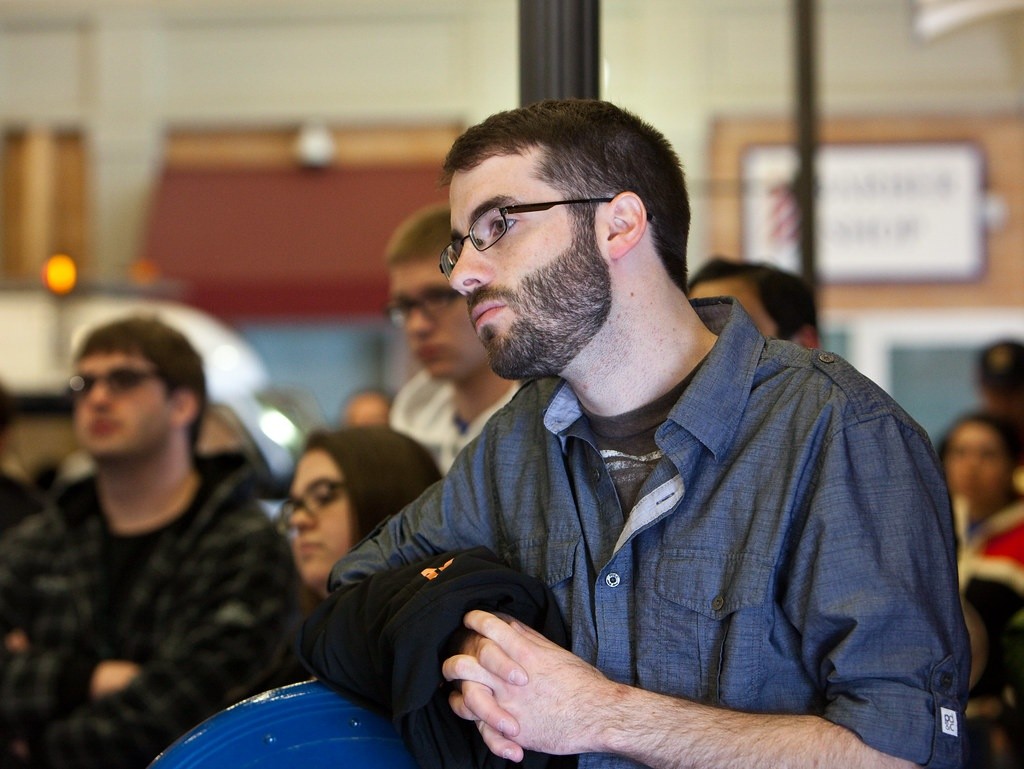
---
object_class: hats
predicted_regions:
[977,339,1023,394]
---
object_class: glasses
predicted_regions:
[385,285,467,328]
[440,197,653,279]
[62,366,159,399]
[280,478,349,527]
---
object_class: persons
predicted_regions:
[0,98,1023,769]
